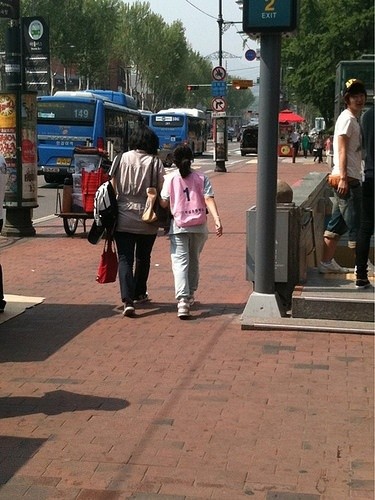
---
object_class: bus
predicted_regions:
[35,88,156,181]
[148,107,212,157]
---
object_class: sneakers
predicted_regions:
[355,260,374,275]
[318,258,349,274]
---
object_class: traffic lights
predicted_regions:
[235,86,248,90]
[186,86,199,91]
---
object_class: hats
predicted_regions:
[344,78,365,95]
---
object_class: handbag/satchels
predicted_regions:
[96,238,119,284]
[142,156,169,228]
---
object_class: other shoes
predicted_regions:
[122,294,148,315]
[177,294,195,317]
[355,278,370,288]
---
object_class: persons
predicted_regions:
[107,127,171,314]
[158,144,222,317]
[354,106,374,290]
[300,131,333,163]
[289,130,300,153]
[320,79,374,277]
[0,155,7,315]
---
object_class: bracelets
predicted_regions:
[213,216,219,220]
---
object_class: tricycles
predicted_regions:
[52,176,109,237]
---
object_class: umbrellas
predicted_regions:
[279,108,304,122]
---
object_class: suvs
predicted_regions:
[228,125,258,156]
